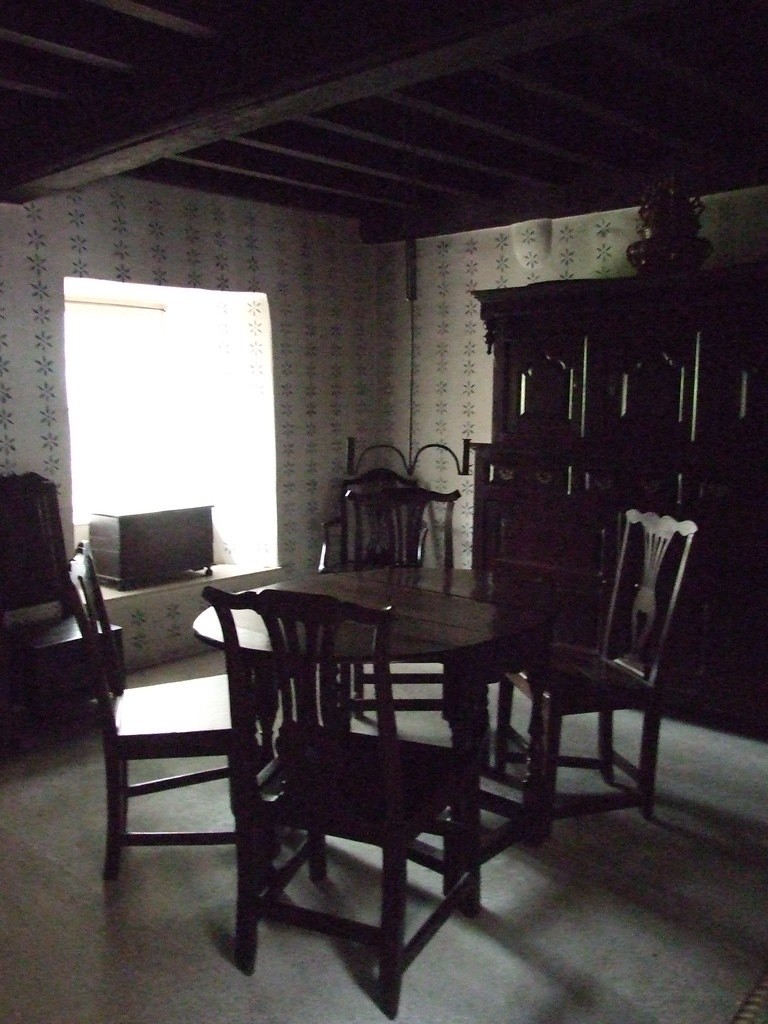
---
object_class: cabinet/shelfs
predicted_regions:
[87,501,216,593]
[467,263,768,744]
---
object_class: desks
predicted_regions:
[190,566,570,895]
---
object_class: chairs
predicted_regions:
[59,538,237,877]
[0,471,127,733]
[315,466,427,575]
[334,483,461,720]
[198,580,487,1021]
[498,507,699,841]
